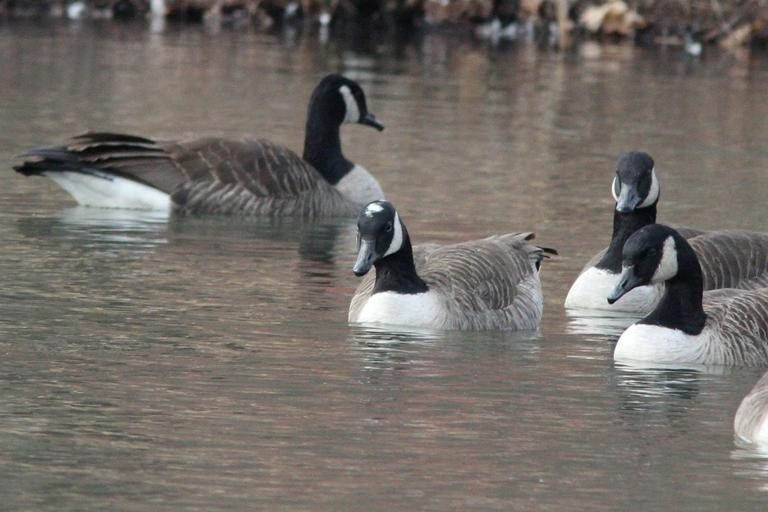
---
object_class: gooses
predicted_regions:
[563,149,768,363]
[12,72,389,217]
[344,197,558,332]
